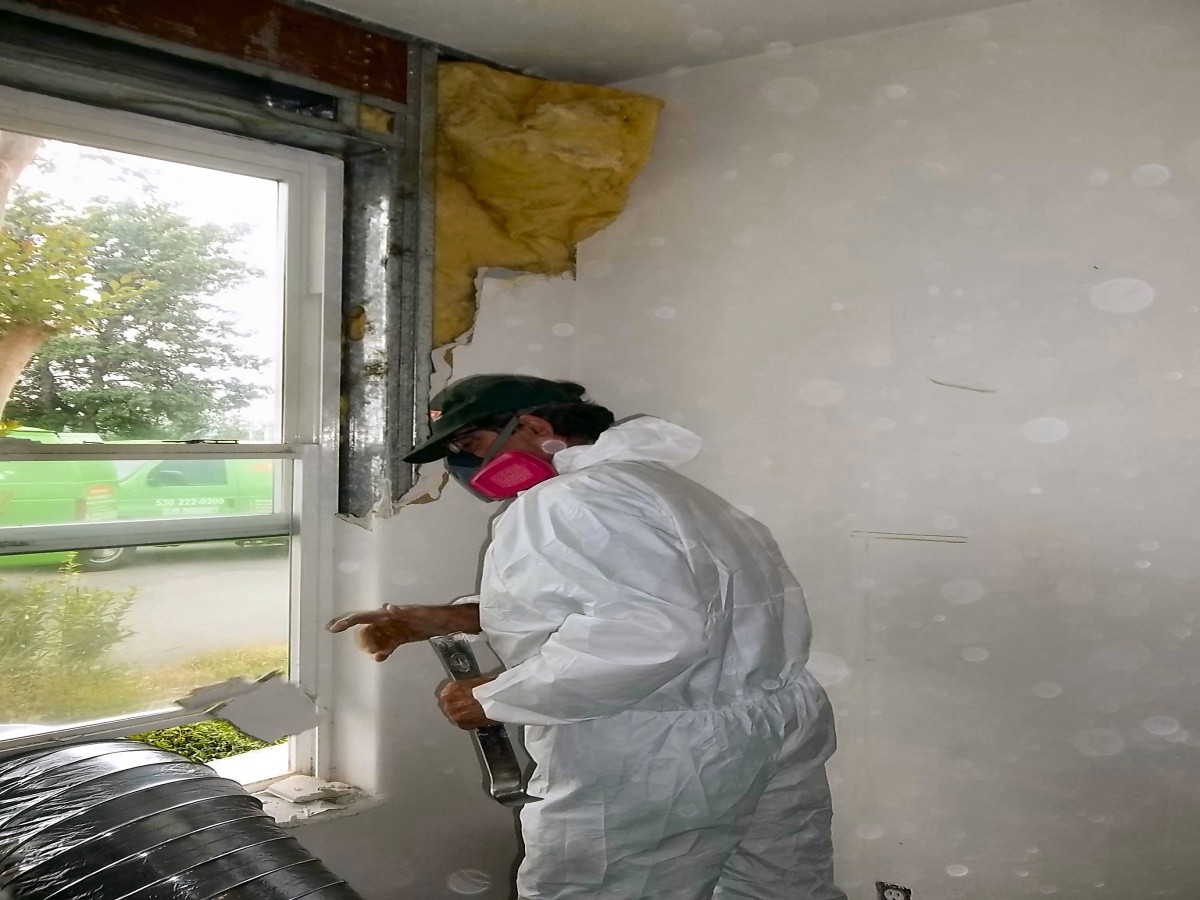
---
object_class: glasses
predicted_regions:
[445,431,474,454]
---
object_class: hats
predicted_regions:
[401,375,581,464]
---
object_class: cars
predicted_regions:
[0,423,288,570]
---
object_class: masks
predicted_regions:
[442,418,522,504]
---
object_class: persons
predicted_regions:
[326,374,834,900]
